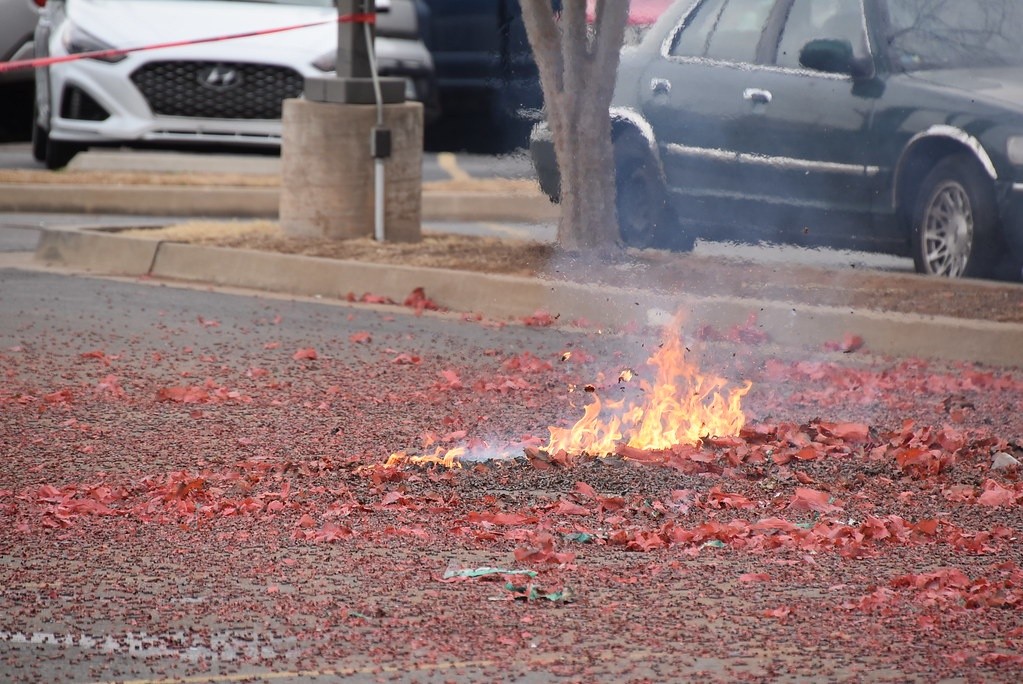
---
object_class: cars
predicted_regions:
[529,0,1023,282]
[1,0,47,137]
[32,0,411,172]
